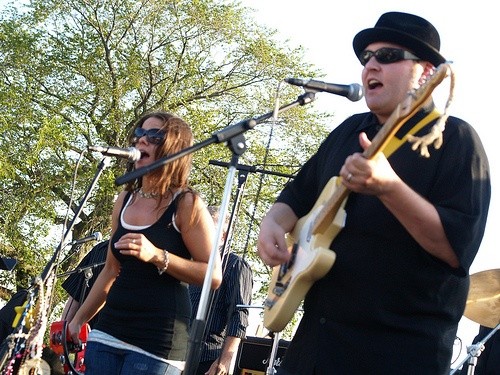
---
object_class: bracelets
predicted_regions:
[157,249,169,275]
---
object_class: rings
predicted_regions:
[132,238,135,243]
[346,173,353,182]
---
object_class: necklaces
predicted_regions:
[139,186,160,199]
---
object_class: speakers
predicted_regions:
[235,336,291,375]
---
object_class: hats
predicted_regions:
[352,12,446,68]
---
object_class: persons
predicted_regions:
[182,206,253,375]
[256,11,491,375]
[65,112,223,375]
[59,240,122,329]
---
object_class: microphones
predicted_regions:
[86,144,141,162]
[69,231,103,245]
[283,77,364,102]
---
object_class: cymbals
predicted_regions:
[50,320,91,375]
[463,268,500,330]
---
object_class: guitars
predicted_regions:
[262,64,448,333]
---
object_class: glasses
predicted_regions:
[359,47,420,64]
[130,127,167,146]
